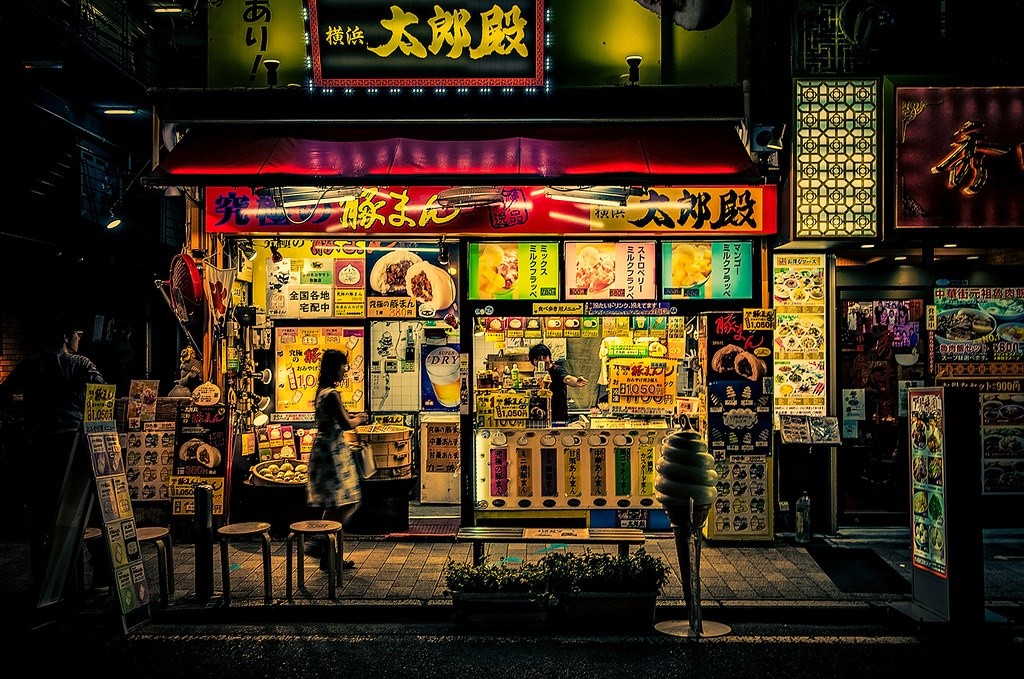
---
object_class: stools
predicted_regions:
[286,520,343,601]
[136,527,174,609]
[216,522,273,603]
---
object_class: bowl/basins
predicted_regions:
[934,298,1024,350]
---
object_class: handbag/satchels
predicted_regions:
[347,442,377,479]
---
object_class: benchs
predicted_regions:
[455,526,646,567]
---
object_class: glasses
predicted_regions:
[72,329,83,337]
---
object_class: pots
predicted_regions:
[252,459,308,485]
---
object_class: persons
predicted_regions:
[0,323,107,568]
[529,344,588,422]
[307,348,368,572]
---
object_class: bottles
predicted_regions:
[502,366,511,388]
[511,364,519,388]
[795,491,811,543]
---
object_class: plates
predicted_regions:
[911,420,944,550]
[983,394,1024,492]
[774,270,824,303]
[778,318,823,348]
[775,362,824,397]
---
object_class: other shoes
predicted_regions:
[302,541,323,558]
[319,558,355,571]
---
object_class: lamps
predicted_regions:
[270,245,283,264]
[626,55,643,86]
[241,369,272,384]
[251,405,268,426]
[436,237,449,265]
[247,392,270,411]
[238,243,257,261]
[102,209,122,229]
[263,60,281,88]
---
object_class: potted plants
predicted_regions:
[443,548,670,634]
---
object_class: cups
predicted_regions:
[636,319,646,328]
[425,346,460,408]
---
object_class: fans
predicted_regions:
[155,254,203,359]
